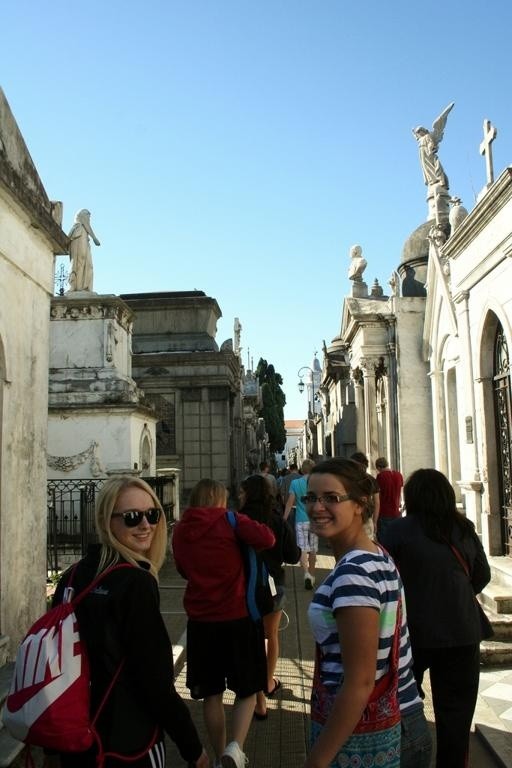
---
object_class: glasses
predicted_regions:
[110,508,161,528]
[300,494,351,504]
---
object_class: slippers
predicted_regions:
[267,678,281,695]
[254,710,269,721]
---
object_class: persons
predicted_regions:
[300,458,434,767]
[234,317,242,358]
[67,209,100,291]
[241,453,404,591]
[411,102,456,222]
[348,245,367,282]
[44,474,210,767]
[171,479,277,768]
[236,473,302,721]
[377,467,491,768]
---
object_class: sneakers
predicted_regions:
[303,573,314,590]
[221,740,247,768]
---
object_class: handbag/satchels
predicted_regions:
[307,669,403,768]
[473,597,496,641]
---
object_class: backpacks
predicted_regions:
[240,542,286,625]
[1,602,95,754]
